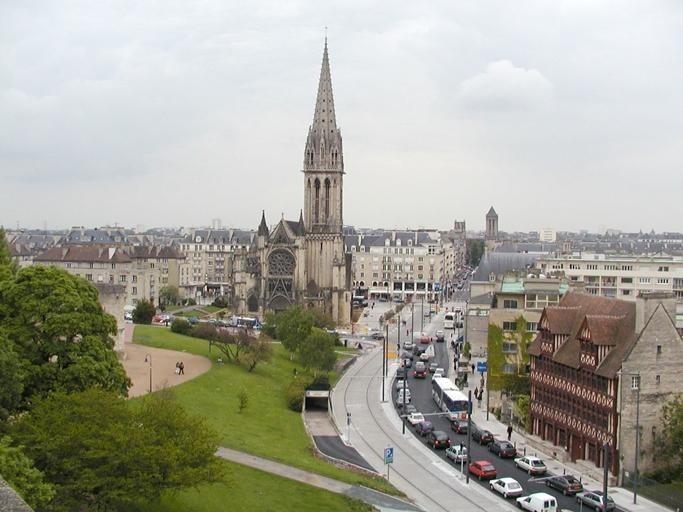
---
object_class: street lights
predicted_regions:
[351,288,434,405]
[143,351,154,395]
[612,369,641,505]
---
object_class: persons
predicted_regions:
[506,423,513,440]
[178,362,185,375]
[461,360,485,408]
[175,360,180,374]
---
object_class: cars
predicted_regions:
[124,310,351,341]
[391,261,478,464]
[464,423,618,512]
